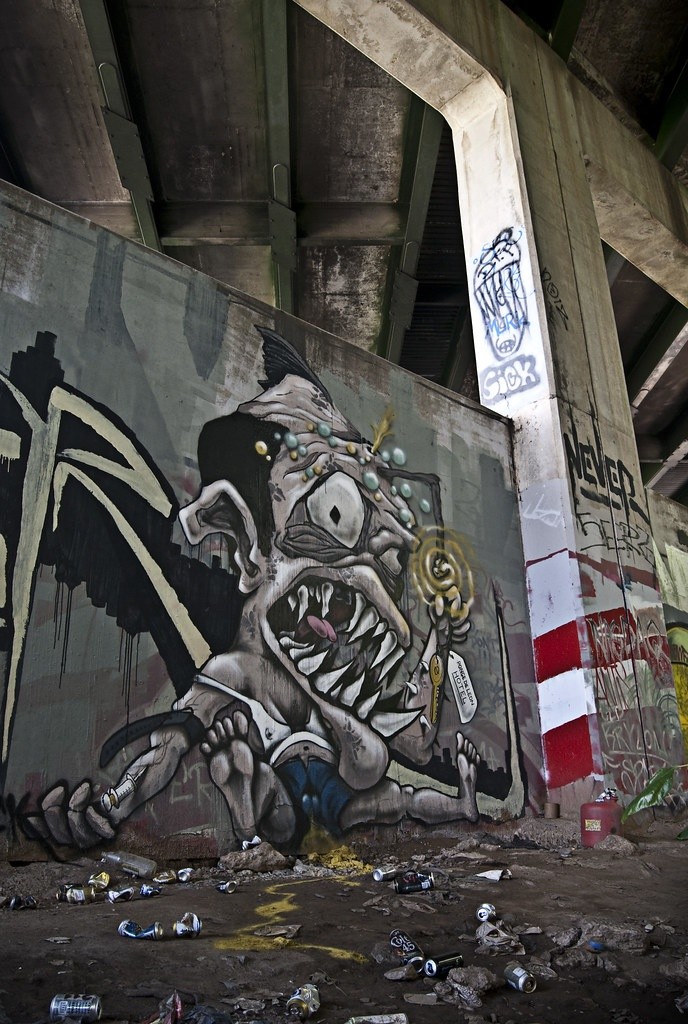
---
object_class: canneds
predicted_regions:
[0,836,536,1024]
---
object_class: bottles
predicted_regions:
[101,850,158,880]
